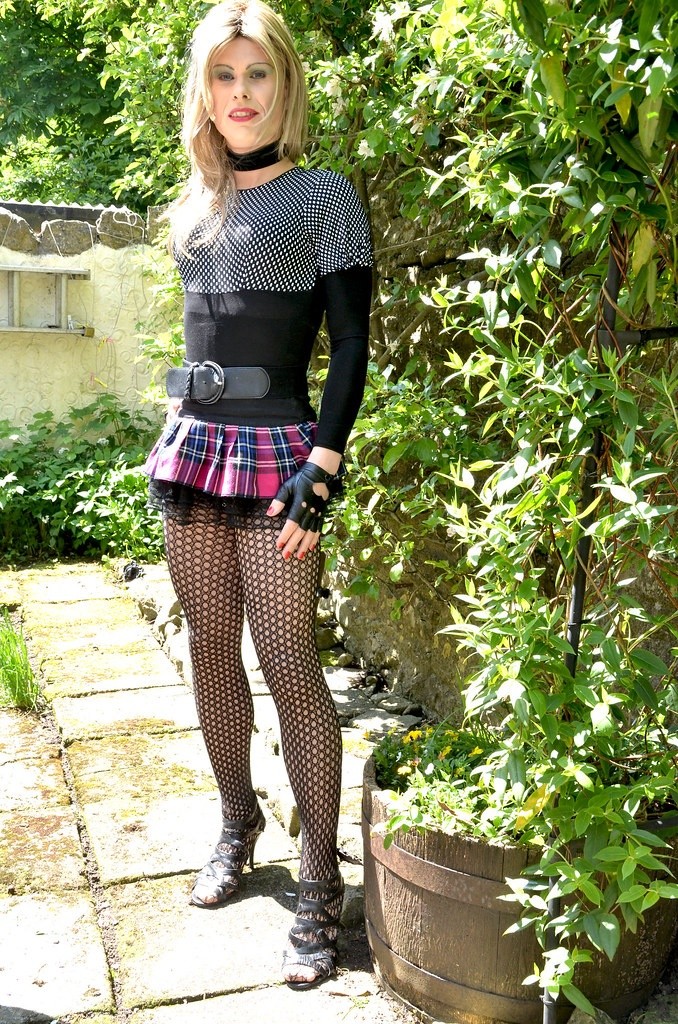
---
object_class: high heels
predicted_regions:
[282,872,345,989]
[190,790,265,908]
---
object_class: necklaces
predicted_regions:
[224,138,290,172]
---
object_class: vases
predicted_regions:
[360,751,678,1024]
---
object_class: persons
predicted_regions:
[142,0,375,993]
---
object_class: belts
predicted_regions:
[166,358,306,405]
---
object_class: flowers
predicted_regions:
[360,722,510,850]
